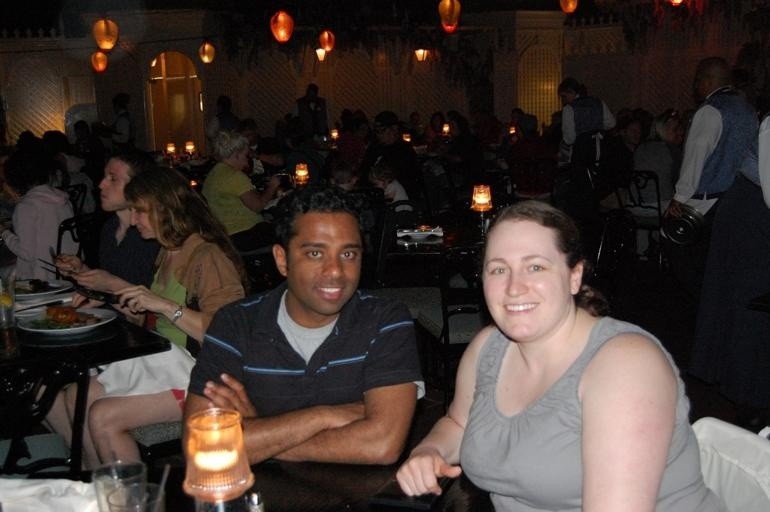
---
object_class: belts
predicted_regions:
[685,193,719,202]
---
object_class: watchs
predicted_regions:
[170,305,185,325]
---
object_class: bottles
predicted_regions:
[0,280,13,330]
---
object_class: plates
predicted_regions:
[14,280,74,300]
[13,294,76,316]
[397,228,443,239]
[17,308,118,335]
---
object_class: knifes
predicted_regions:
[14,301,64,313]
[77,288,132,305]
[37,256,79,275]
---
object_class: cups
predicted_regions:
[107,483,165,512]
[93,460,146,512]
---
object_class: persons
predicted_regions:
[29,145,155,464]
[687,113,770,434]
[181,184,426,466]
[395,199,728,512]
[1,58,769,301]
[66,165,252,471]
